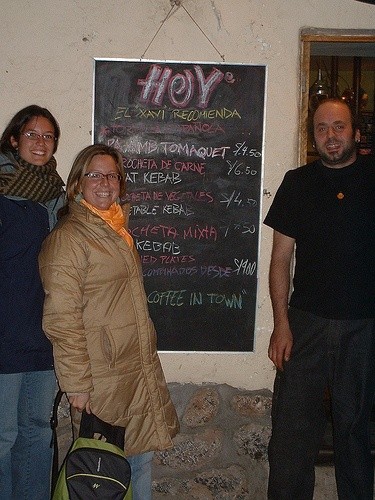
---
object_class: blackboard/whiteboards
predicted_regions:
[90,59,269,356]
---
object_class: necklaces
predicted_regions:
[337,191,345,198]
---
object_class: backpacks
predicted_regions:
[50,388,133,500]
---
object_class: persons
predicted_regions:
[263,95,375,500]
[0,105,65,500]
[38,143,182,500]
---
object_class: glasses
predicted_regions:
[18,129,57,143]
[80,171,122,182]
